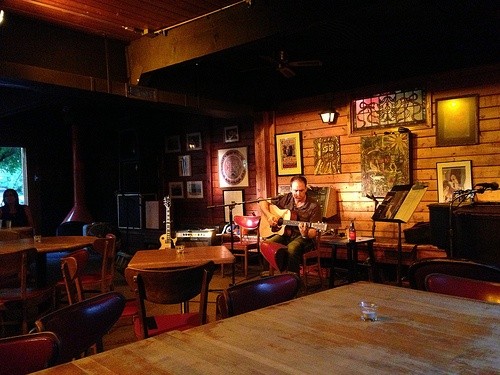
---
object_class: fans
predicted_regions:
[243,47,323,78]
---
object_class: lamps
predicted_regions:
[318,101,337,125]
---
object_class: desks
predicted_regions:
[117,227,215,247]
[428,200,500,266]
[315,234,379,286]
[0,237,37,335]
[126,246,237,314]
[24,329,288,375]
[181,280,500,375]
[31,236,97,253]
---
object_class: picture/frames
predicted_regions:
[187,180,204,198]
[176,154,193,177]
[216,145,250,188]
[186,131,202,151]
[223,124,239,142]
[168,181,184,199]
[223,189,245,222]
[435,93,479,146]
[436,160,473,203]
[165,134,181,154]
[274,131,303,176]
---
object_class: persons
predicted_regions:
[0,188,34,279]
[269,174,322,279]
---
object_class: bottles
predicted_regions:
[349,222,356,242]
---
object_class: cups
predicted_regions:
[358,301,379,322]
[0,220,3,229]
[6,220,11,228]
[34,234,41,243]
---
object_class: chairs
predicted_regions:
[0,221,216,375]
[267,230,324,292]
[220,214,262,279]
[407,257,500,305]
[216,272,303,321]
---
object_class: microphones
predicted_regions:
[277,193,287,200]
[365,193,379,205]
[475,181,499,190]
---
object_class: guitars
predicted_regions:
[258,203,328,238]
[158,195,178,250]
